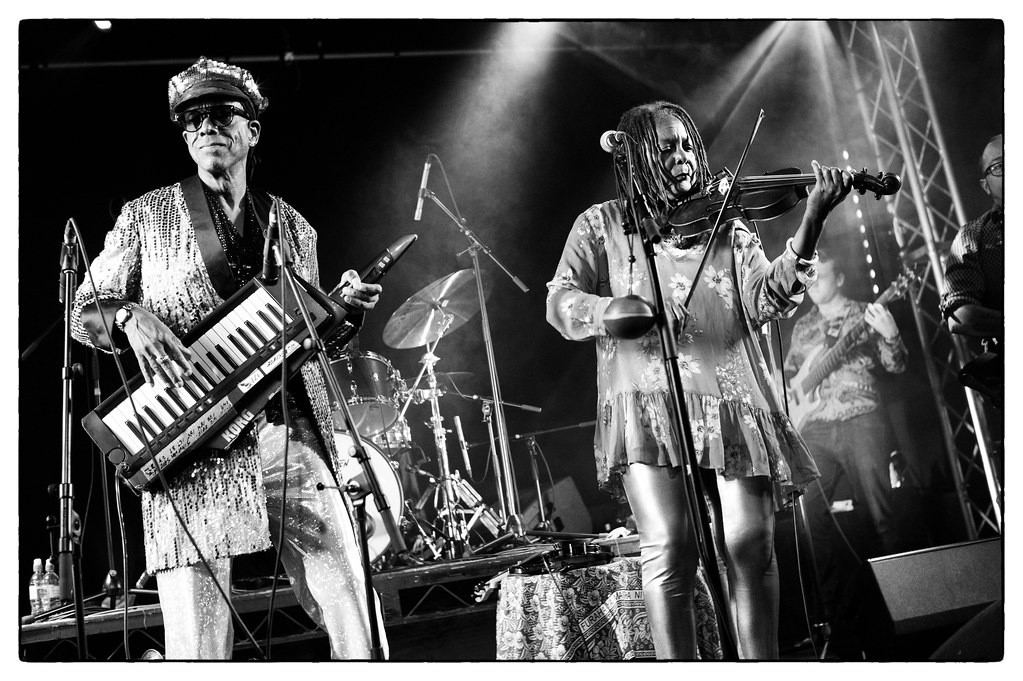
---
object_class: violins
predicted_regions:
[669,166,901,240]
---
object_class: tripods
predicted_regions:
[425,190,614,557]
[20,346,163,623]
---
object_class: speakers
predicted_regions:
[847,534,1002,663]
[487,475,593,542]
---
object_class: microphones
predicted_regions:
[404,464,435,478]
[413,156,431,221]
[59,218,78,304]
[260,202,280,285]
[600,130,626,153]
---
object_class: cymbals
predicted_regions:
[381,268,494,350]
[404,367,476,390]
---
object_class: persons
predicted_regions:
[937,134,1002,405]
[547,102,852,659]
[777,246,908,619]
[69,56,391,661]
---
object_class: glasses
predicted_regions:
[174,104,251,133]
[981,161,1003,179]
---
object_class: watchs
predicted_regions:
[115,302,138,331]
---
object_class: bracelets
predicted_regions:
[884,331,900,341]
[786,237,819,266]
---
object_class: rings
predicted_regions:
[155,355,169,365]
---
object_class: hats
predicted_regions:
[168,55,269,127]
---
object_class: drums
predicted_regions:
[318,430,402,568]
[372,414,414,458]
[321,352,398,434]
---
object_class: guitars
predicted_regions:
[772,261,927,437]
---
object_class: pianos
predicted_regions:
[89,234,417,490]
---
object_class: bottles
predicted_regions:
[38,564,59,612]
[29,558,45,614]
